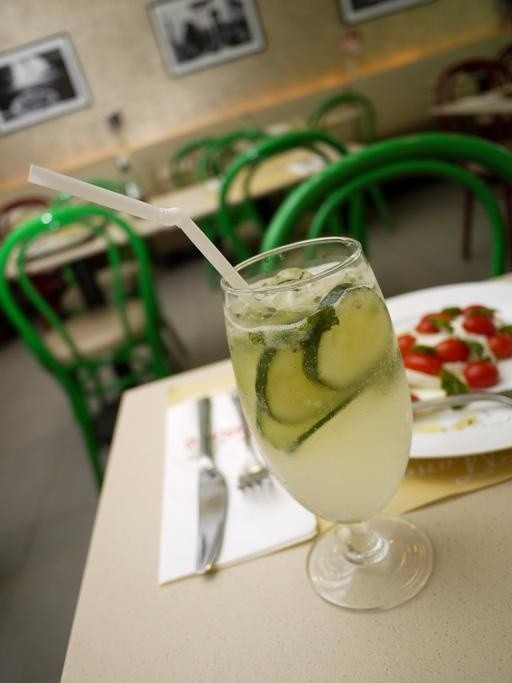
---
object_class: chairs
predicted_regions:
[216,30,512,300]
[0,30,220,511]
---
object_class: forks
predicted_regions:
[228,378,272,500]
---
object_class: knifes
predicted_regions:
[189,397,230,573]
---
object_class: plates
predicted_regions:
[382,279,511,463]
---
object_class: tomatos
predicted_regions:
[396,305,512,389]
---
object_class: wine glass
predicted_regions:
[220,235,438,615]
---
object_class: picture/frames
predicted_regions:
[142,0,271,80]
[328,0,434,27]
